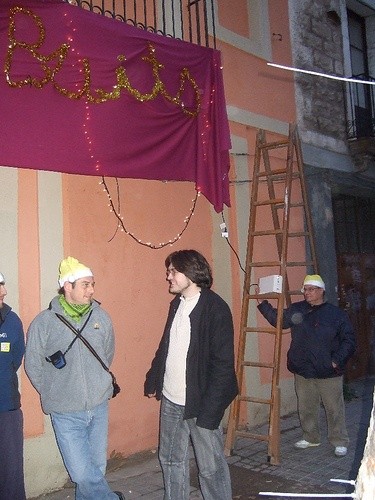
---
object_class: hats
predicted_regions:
[300,275,326,294]
[0,272,5,284]
[59,256,93,288]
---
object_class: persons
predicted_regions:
[256,276,357,456]
[142,250,239,500]
[26,258,126,500]
[0,272,25,500]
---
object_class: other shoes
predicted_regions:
[113,491,125,500]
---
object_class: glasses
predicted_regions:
[303,287,321,292]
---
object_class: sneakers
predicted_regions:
[335,446,347,456]
[295,439,320,448]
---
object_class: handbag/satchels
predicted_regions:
[109,371,120,398]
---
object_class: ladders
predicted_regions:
[216,120,320,466]
